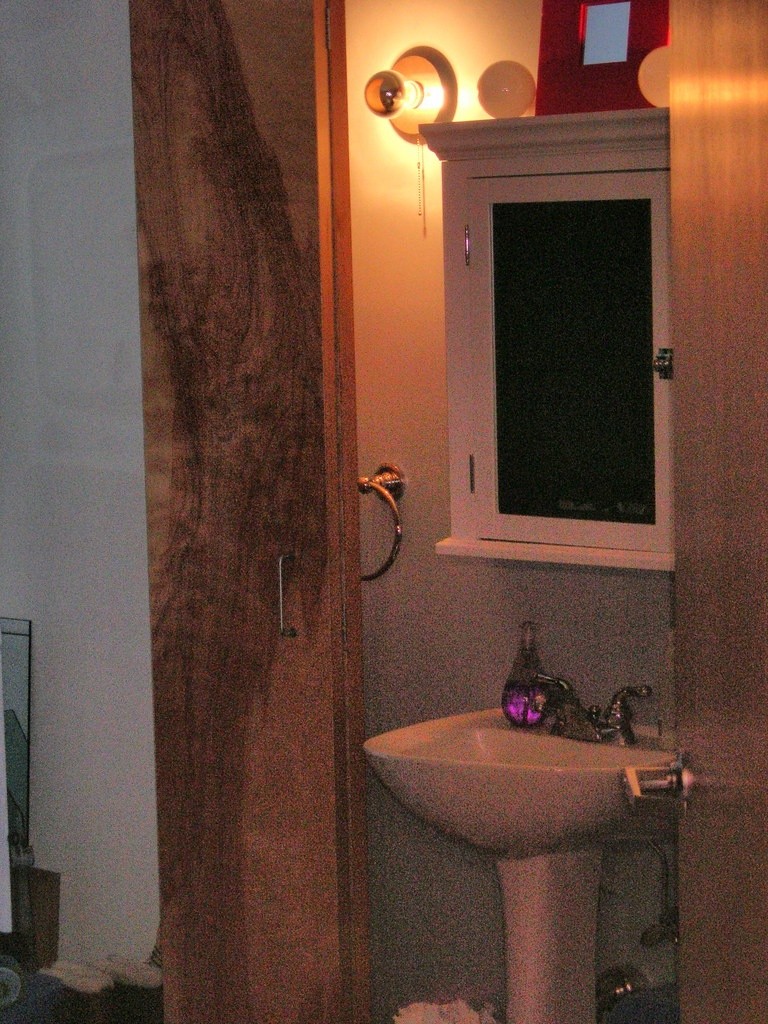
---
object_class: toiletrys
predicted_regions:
[499,616,549,729]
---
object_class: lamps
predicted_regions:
[364,46,458,145]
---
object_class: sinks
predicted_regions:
[363,703,682,854]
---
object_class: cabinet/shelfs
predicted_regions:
[416,106,676,575]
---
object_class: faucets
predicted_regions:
[556,697,603,742]
